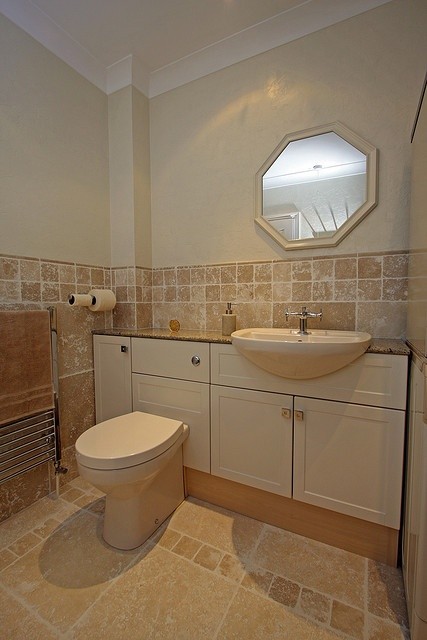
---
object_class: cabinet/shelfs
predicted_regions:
[131,337,212,503]
[92,335,132,426]
[209,343,408,569]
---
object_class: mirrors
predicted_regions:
[255,122,379,251]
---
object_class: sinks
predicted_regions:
[230,328,372,379]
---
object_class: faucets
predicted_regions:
[285,307,322,334]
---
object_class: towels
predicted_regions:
[0,310,54,425]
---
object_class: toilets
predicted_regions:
[75,411,190,551]
